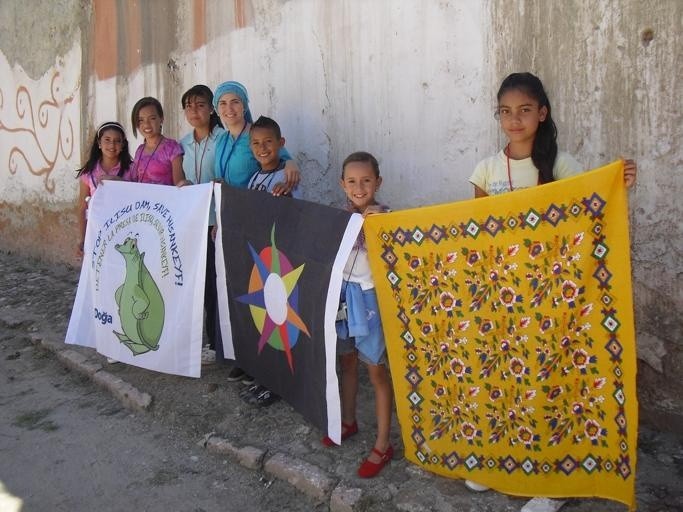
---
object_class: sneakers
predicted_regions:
[228,368,278,407]
[521,496,565,511]
[201,342,217,366]
[323,420,358,446]
[358,444,393,477]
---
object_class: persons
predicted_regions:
[213,80,301,382]
[98,97,192,185]
[74,119,136,257]
[319,151,393,479]
[462,71,637,512]
[210,113,305,410]
[179,81,225,366]
